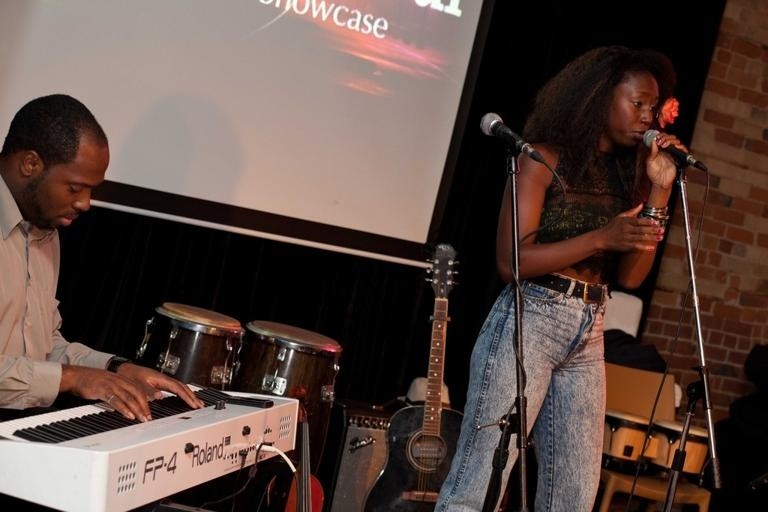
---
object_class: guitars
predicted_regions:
[362,244,465,511]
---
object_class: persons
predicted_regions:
[0,91,206,512]
[638,96,681,211]
[433,42,691,512]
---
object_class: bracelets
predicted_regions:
[642,204,670,231]
[107,360,128,374]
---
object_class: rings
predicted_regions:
[106,393,116,403]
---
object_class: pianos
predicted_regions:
[0,385,301,510]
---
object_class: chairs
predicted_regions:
[599,362,713,512]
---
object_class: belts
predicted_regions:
[525,274,609,305]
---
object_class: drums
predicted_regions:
[234,319,344,472]
[602,411,652,474]
[136,300,244,391]
[647,419,708,488]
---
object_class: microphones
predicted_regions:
[642,129,711,172]
[480,111,547,166]
